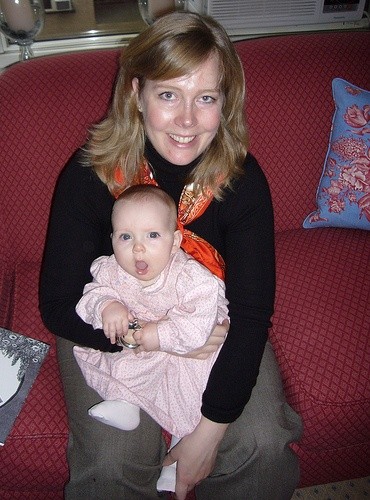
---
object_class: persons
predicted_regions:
[38,10,304,500]
[73,184,230,492]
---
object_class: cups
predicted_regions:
[139,0,185,29]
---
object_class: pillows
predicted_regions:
[302,77,370,233]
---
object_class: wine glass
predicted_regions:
[0,0,45,59]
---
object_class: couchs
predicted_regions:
[0,23,370,500]
